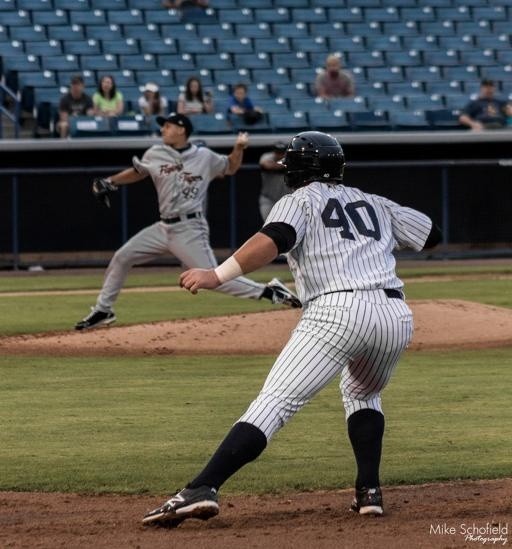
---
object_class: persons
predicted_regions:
[315,56,355,99]
[458,78,512,129]
[92,75,124,117]
[176,78,213,113]
[54,75,93,138]
[225,84,254,125]
[258,142,294,223]
[138,82,169,116]
[142,130,443,527]
[75,112,302,331]
[162,0,208,8]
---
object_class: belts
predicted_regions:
[383,289,402,299]
[160,212,200,224]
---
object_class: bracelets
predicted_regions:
[214,255,243,284]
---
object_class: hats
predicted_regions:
[156,112,192,130]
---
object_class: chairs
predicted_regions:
[0,0,512,136]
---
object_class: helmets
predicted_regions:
[277,131,347,187]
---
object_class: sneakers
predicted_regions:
[142,486,219,527]
[75,310,116,330]
[351,487,384,515]
[267,279,302,308]
